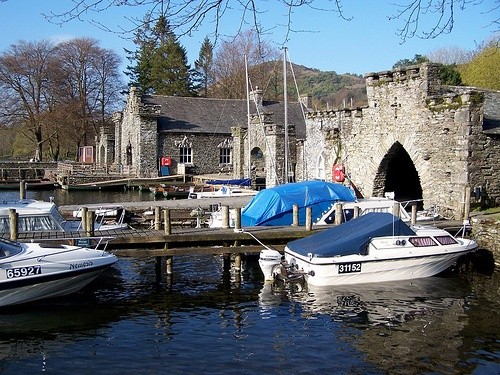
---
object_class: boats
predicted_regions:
[147,182,214,196]
[0,202,129,233]
[0,237,118,307]
[234,177,357,227]
[258,212,479,286]
[188,186,260,199]
[314,198,444,225]
